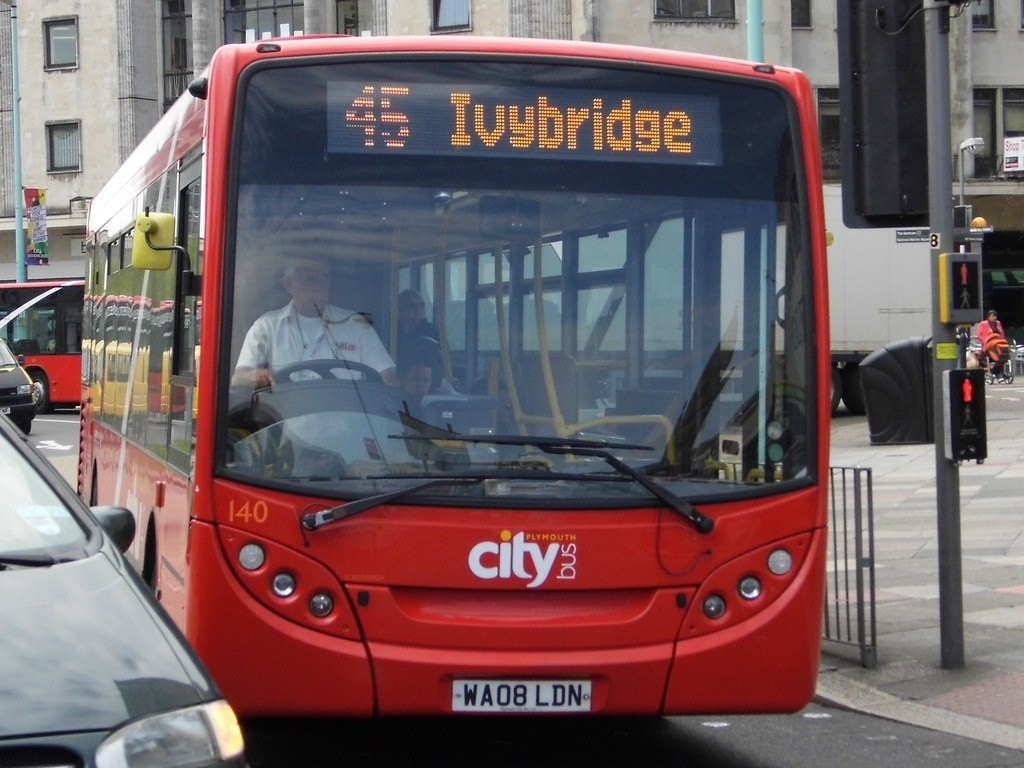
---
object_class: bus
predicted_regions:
[0,281,86,412]
[77,34,835,723]
[82,292,203,420]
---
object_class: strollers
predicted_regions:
[976,333,1014,385]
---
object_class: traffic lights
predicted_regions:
[942,368,988,464]
[937,253,984,324]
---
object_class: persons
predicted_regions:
[397,287,447,389]
[229,254,403,392]
[976,309,1006,346]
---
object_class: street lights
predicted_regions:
[953,136,985,368]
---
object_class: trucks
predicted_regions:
[419,181,933,421]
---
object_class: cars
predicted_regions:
[0,411,251,768]
[1,339,36,435]
